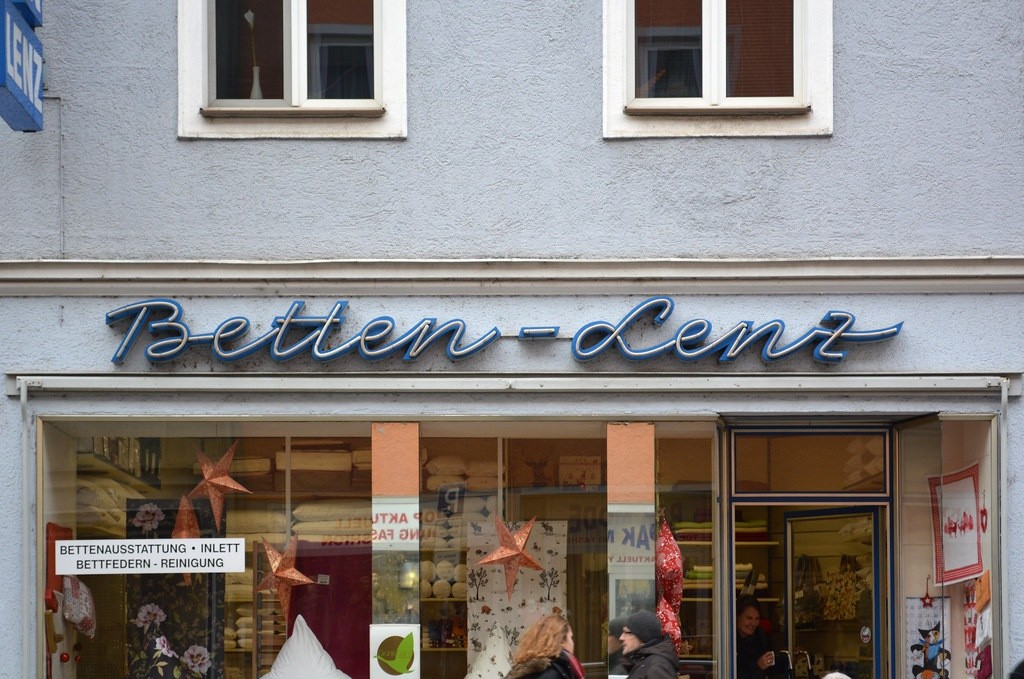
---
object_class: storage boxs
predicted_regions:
[558,456,601,486]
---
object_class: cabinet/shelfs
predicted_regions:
[253,540,287,679]
[224,492,499,652]
[675,542,780,658]
[76,452,162,538]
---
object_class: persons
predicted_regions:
[618,611,680,679]
[506,607,586,679]
[737,594,775,679]
[608,620,632,675]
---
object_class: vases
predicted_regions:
[250,66,263,99]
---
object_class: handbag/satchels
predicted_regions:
[776,553,867,632]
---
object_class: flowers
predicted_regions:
[244,9,256,66]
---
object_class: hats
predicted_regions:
[609,618,626,637]
[624,611,660,642]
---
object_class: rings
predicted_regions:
[768,660,770,663]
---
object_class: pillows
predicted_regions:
[833,435,884,487]
[656,520,684,657]
[292,496,505,548]
[463,623,515,679]
[258,614,353,679]
[76,476,146,531]
[63,575,96,639]
[426,455,506,491]
[224,603,287,649]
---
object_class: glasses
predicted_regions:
[622,630,633,635]
